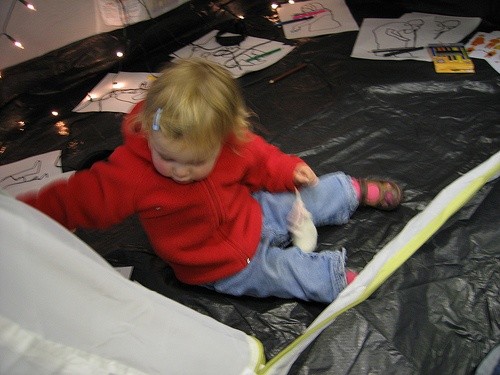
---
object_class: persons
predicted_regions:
[16,58,403,303]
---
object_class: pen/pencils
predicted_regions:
[367,46,424,56]
[272,9,332,27]
[245,48,282,61]
[269,63,308,83]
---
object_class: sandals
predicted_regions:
[357,177,402,210]
[347,265,384,299]
